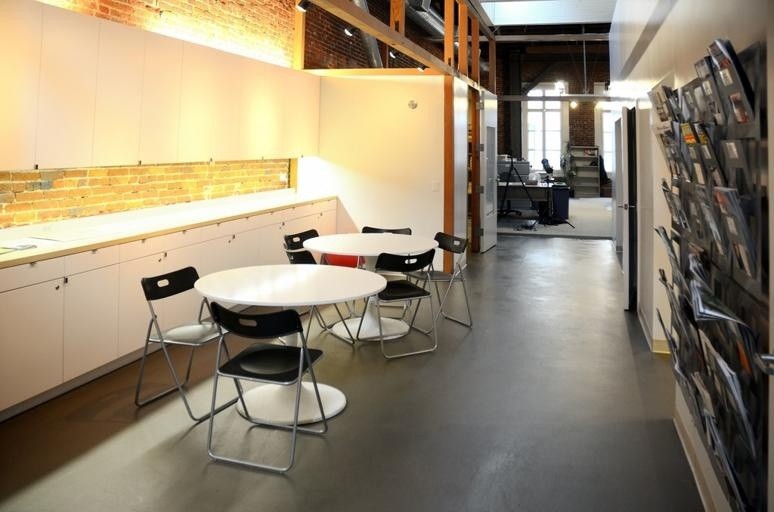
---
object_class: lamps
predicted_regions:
[297,0,426,71]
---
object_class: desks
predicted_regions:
[496,181,554,217]
[195,262,389,427]
[303,233,438,341]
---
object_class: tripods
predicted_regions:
[526,175,575,230]
[498,147,539,225]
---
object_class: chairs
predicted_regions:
[400,232,472,334]
[357,248,437,360]
[352,226,413,321]
[209,301,325,476]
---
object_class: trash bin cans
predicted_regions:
[552,182,571,219]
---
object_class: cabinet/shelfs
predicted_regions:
[0,193,338,424]
[540,181,568,220]
[569,146,601,199]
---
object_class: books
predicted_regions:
[646,38,765,510]
